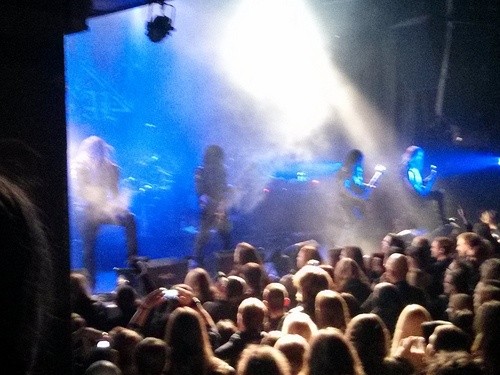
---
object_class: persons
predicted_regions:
[401,145,449,223]
[237,345,291,375]
[298,327,364,375]
[0,178,53,375]
[332,149,379,233]
[346,313,409,375]
[167,308,235,375]
[71,207,500,375]
[188,145,234,271]
[74,134,137,290]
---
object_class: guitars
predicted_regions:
[339,165,386,221]
[422,166,438,195]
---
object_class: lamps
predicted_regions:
[144,0,176,43]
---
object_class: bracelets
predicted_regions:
[196,300,200,303]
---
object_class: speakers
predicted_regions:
[213,247,264,274]
[146,257,187,288]
